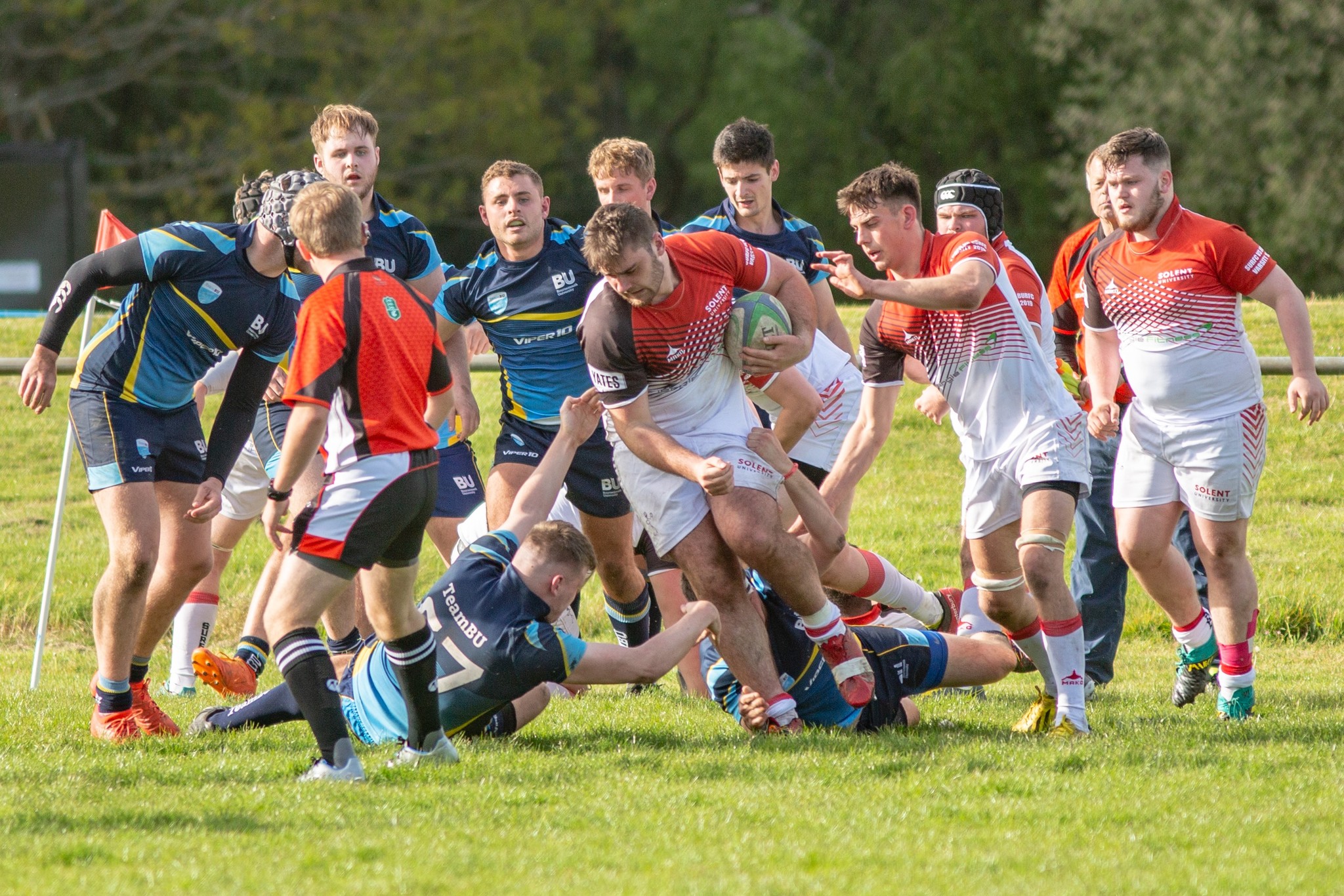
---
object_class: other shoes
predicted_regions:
[970,629,1036,674]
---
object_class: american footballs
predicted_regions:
[719,290,793,371]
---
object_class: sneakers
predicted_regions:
[1216,686,1255,721]
[191,647,259,705]
[1172,624,1219,707]
[156,678,195,701]
[294,738,365,783]
[88,670,184,747]
[926,588,965,636]
[764,715,802,736]
[1013,684,1090,743]
[386,723,460,768]
[817,621,874,709]
[185,705,228,734]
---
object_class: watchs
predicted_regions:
[267,477,293,501]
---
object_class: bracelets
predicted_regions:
[781,462,798,480]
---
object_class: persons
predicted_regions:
[21,168,331,740]
[786,161,1092,744]
[259,178,454,792]
[923,167,1068,653]
[1067,125,1333,725]
[1032,140,1258,694]
[169,104,1023,781]
